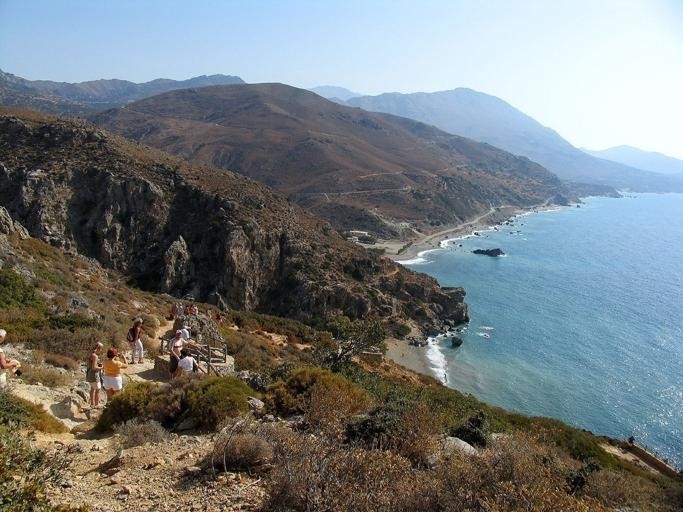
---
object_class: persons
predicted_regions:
[0,328,20,393]
[85,341,105,408]
[171,302,221,322]
[618,439,633,450]
[180,325,190,341]
[168,329,192,380]
[173,347,199,379]
[128,318,145,364]
[102,346,128,404]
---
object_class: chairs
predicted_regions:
[90,402,102,409]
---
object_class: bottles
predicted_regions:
[176,330,182,334]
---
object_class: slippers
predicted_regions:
[126,325,138,342]
